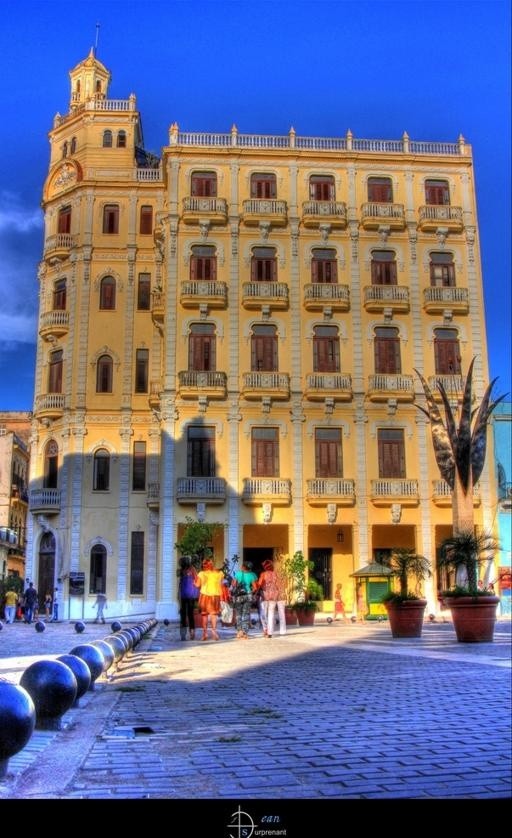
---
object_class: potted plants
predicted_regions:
[283,551,324,627]
[380,527,500,644]
[173,516,240,628]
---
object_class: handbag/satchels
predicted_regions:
[16,608,21,619]
[230,570,246,597]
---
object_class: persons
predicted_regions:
[20,582,38,624]
[258,560,279,638]
[354,581,367,625]
[478,579,484,592]
[43,590,52,623]
[53,588,59,620]
[487,582,495,595]
[15,588,25,620]
[333,583,350,624]
[32,594,40,622]
[91,588,108,624]
[273,560,288,636]
[228,561,260,640]
[4,585,19,625]
[178,556,199,640]
[191,557,225,640]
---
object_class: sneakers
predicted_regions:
[189,628,272,640]
